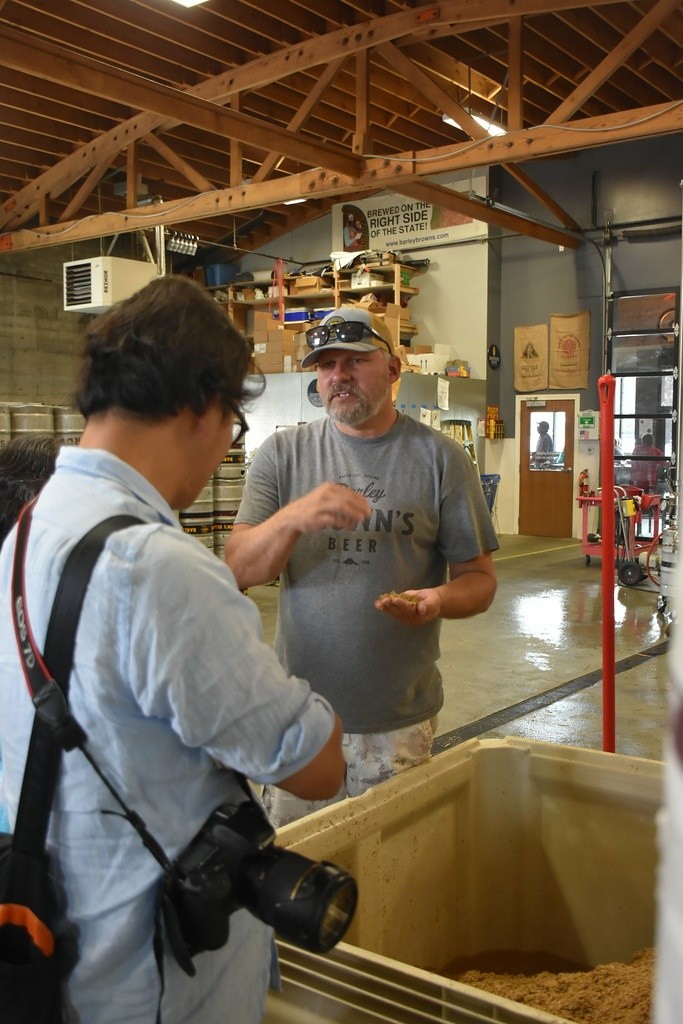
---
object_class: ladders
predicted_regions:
[449,420,480,477]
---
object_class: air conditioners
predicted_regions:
[62,255,157,315]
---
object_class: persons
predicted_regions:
[614,440,625,464]
[630,434,670,497]
[342,212,364,253]
[226,308,497,844]
[536,420,554,468]
[0,275,347,1024]
[0,437,71,555]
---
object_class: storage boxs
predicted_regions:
[401,347,413,365]
[254,310,316,375]
[294,276,333,294]
[350,273,384,289]
[236,285,288,301]
[413,343,450,372]
[289,280,296,295]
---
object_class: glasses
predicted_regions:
[305,321,392,354]
[225,400,249,447]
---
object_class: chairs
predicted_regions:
[480,473,502,537]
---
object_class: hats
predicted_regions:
[301,307,393,368]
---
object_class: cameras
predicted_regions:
[159,799,360,961]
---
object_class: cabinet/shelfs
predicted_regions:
[205,263,420,347]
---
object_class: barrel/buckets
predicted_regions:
[0,401,89,446]
[166,450,247,562]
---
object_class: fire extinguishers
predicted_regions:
[578,468,590,508]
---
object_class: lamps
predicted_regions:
[442,63,507,136]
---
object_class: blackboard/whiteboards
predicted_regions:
[481,473,500,514]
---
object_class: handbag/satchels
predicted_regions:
[0,830,61,1024]
